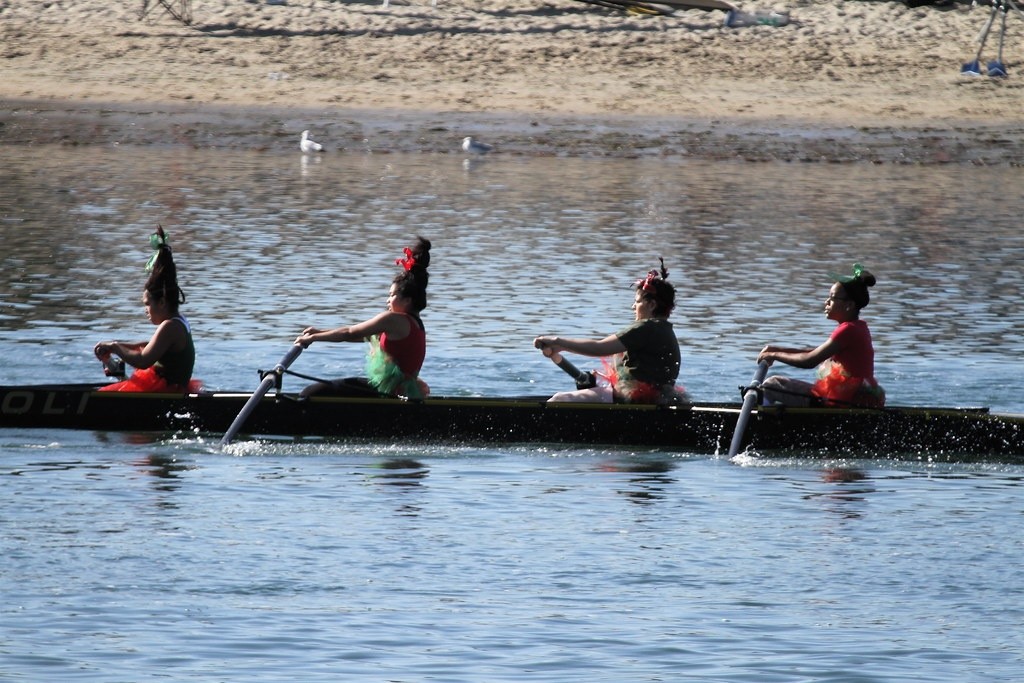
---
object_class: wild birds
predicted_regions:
[300,129,326,152]
[458,137,492,160]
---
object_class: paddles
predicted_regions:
[219,331,313,445]
[727,360,768,462]
[538,338,596,389]
[97,347,130,382]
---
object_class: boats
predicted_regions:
[0,374,1024,459]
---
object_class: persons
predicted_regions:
[293,238,431,397]
[533,257,681,404]
[756,272,886,408]
[94,226,195,395]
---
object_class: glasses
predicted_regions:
[829,294,854,305]
